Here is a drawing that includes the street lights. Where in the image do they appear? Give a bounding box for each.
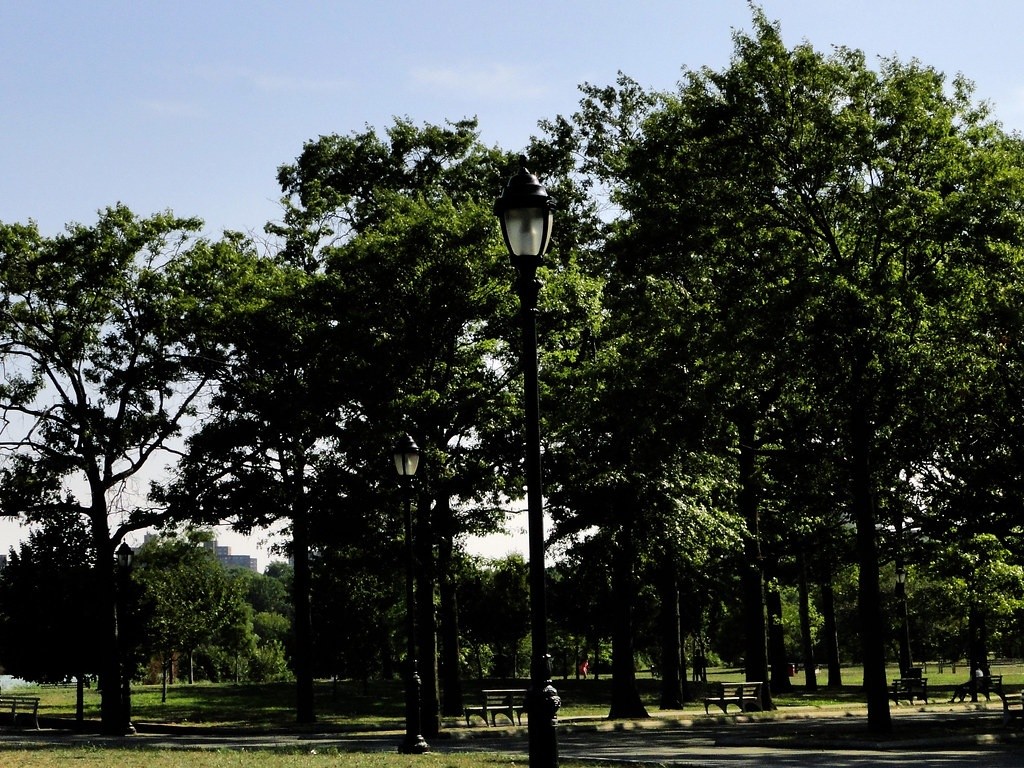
[391,429,431,755]
[491,152,563,768]
[894,566,913,678]
[116,542,137,737]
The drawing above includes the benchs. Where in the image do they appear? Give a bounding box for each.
[888,678,928,706]
[700,681,764,714]
[464,688,529,728]
[0,697,41,732]
[961,674,1004,703]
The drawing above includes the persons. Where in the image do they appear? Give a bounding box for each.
[692,649,707,681]
[580,660,588,679]
[948,662,990,703]
